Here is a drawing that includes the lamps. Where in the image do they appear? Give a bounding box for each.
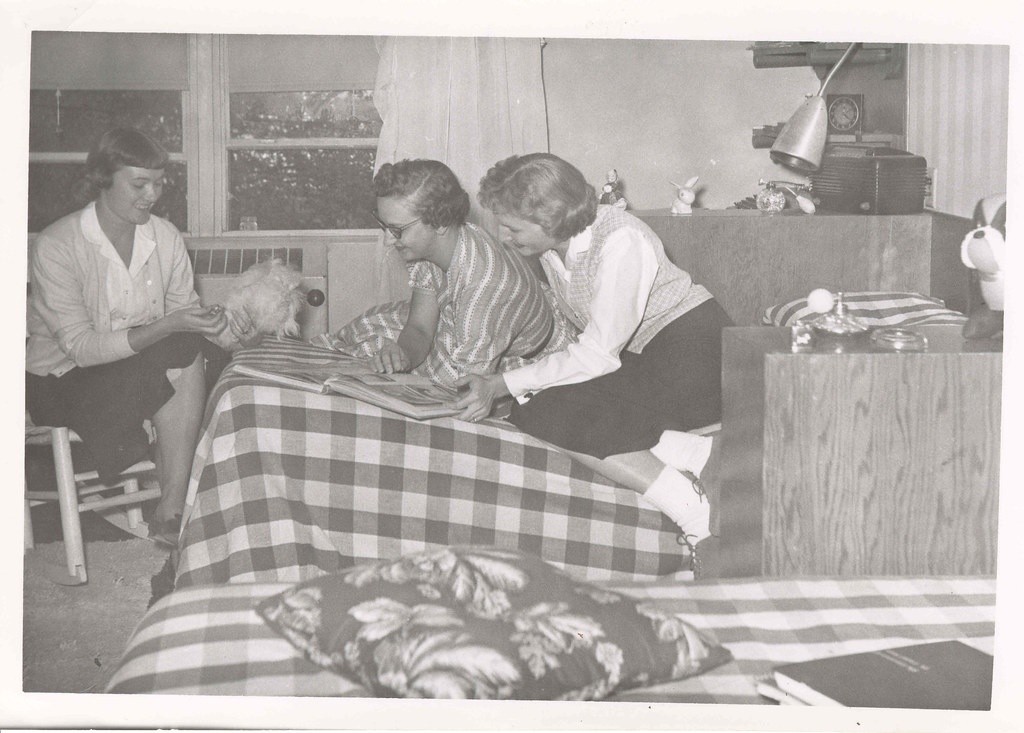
[770,41,861,174]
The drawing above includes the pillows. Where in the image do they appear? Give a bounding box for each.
[250,541,731,699]
[764,297,949,352]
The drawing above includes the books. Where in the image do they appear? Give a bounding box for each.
[758,638,992,711]
[232,358,466,418]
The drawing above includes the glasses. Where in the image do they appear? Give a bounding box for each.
[369,208,423,239]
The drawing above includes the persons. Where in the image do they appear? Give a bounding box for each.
[25,123,254,546]
[440,152,737,587]
[309,156,585,424]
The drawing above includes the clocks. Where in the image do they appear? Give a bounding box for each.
[826,92,866,135]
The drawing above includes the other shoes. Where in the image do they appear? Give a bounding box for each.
[693,433,722,537]
[148,514,181,547]
[679,534,723,580]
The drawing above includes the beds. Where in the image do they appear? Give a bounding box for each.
[110,292,998,710]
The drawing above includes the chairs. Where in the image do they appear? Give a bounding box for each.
[25,230,174,588]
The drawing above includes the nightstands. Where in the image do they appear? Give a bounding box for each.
[714,327,999,574]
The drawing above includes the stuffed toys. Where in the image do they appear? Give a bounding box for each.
[204,255,303,354]
[957,189,1007,340]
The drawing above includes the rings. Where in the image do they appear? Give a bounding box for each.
[474,418,477,422]
[242,329,248,334]
